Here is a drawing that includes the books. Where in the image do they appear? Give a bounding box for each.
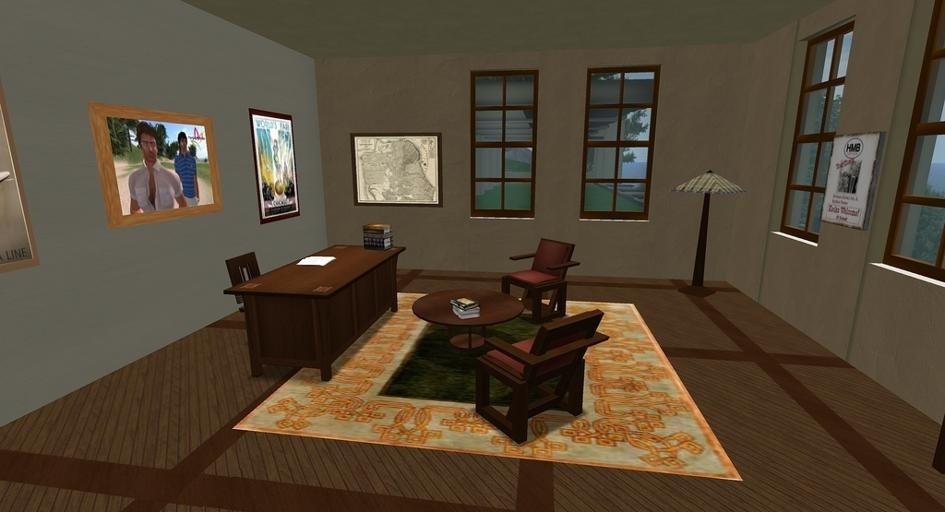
[363,223,393,250]
[295,255,336,266]
[450,296,480,319]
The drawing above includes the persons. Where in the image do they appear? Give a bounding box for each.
[126,121,199,214]
